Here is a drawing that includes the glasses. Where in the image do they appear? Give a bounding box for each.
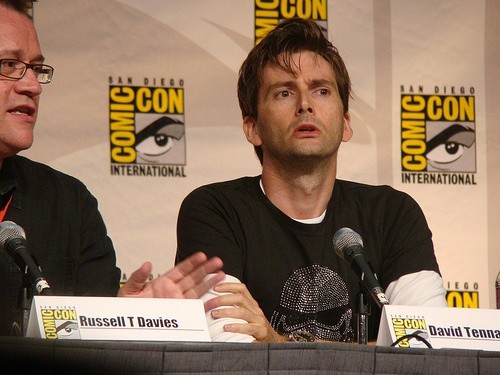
[0,59,54,84]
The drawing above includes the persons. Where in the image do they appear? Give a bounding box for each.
[0,0,226,300]
[176,19,447,344]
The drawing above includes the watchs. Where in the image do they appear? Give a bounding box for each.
[291,329,316,343]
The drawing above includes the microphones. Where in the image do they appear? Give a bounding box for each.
[0,221,52,295]
[332,227,390,309]
